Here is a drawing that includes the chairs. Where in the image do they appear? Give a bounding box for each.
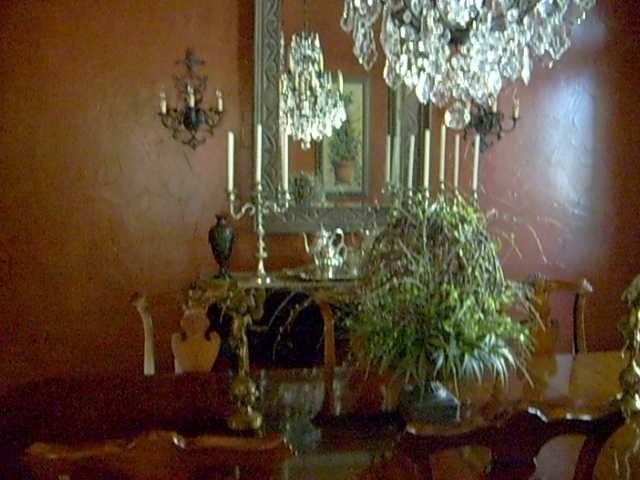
[22,430,296,478]
[132,277,254,373]
[509,275,594,352]
[396,405,625,479]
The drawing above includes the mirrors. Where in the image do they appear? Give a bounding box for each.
[254,0,432,237]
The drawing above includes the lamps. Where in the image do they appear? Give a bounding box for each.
[340,1,596,154]
[158,49,228,149]
[286,0,348,152]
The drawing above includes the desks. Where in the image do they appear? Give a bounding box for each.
[0,351,640,479]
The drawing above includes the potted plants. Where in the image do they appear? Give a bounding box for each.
[272,180,567,426]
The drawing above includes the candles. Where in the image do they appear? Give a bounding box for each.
[280,122,289,191]
[254,122,262,184]
[384,125,480,189]
[227,131,235,191]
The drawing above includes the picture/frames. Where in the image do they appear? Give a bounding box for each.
[315,74,368,198]
